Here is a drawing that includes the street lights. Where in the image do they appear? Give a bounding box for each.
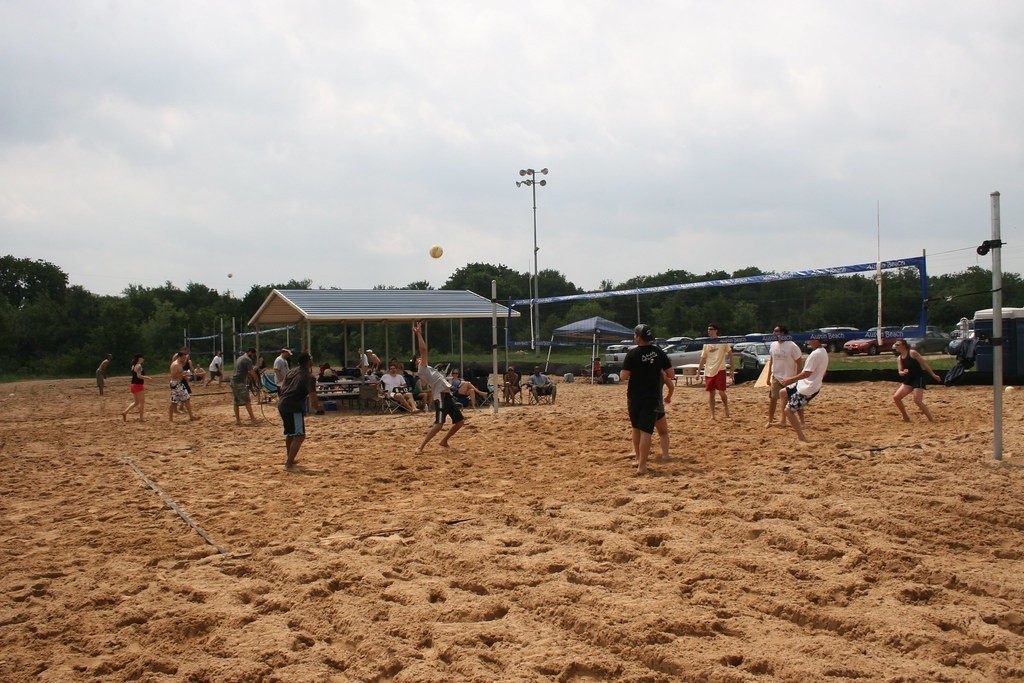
[515,167,549,357]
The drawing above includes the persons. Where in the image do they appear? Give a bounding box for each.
[273,346,293,426]
[769,329,828,443]
[696,323,735,420]
[763,325,802,429]
[96,354,112,395]
[277,352,323,472]
[503,367,518,406]
[356,348,369,371]
[893,340,941,422]
[627,374,674,460]
[409,322,465,455]
[366,357,488,413]
[527,366,556,405]
[122,354,153,422]
[168,347,223,422]
[366,349,381,371]
[620,324,675,474]
[230,348,258,425]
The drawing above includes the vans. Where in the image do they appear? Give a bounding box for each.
[900,324,939,334]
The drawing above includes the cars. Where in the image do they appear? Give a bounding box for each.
[804,327,860,354]
[891,331,950,356]
[843,326,903,354]
[739,341,774,371]
[947,329,975,359]
[605,333,768,368]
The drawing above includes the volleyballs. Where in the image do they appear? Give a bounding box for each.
[324,369,332,376]
[227,274,232,278]
[1005,386,1015,392]
[429,245,443,258]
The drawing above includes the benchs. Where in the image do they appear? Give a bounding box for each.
[316,390,360,409]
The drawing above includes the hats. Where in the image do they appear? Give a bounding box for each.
[282,349,293,355]
[635,324,655,340]
[409,355,418,372]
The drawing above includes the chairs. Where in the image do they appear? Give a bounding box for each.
[497,372,523,406]
[528,372,551,405]
[380,381,411,415]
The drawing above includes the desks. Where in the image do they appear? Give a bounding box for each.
[317,382,379,410]
[674,374,707,386]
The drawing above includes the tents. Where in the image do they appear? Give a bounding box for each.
[544,316,635,385]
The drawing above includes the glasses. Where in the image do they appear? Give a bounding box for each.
[453,373,458,374]
[508,370,513,372]
